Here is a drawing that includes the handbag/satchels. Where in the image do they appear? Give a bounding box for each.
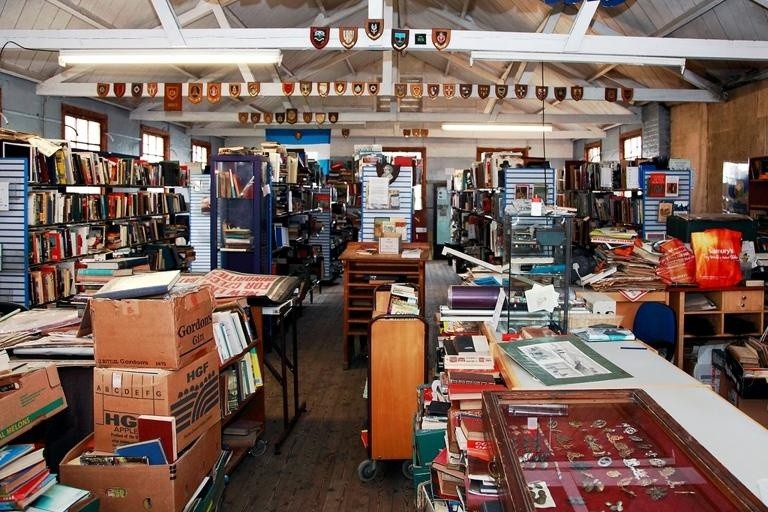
[692,230,743,288]
[659,239,695,286]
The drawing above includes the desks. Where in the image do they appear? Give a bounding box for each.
[478,321,768,511]
[261,282,306,456]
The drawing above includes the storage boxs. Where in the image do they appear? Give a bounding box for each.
[0,365,68,447]
[57,281,222,511]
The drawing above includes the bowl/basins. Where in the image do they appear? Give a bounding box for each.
[653,156,670,170]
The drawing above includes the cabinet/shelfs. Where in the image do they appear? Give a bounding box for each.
[337,242,433,369]
[665,286,768,369]
[450,169,557,275]
[214,305,265,477]
[557,158,691,257]
[0,158,192,309]
[747,154,768,251]
[359,282,430,462]
[211,155,324,304]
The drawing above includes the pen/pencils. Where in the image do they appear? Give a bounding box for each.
[620,347,648,349]
[356,249,373,252]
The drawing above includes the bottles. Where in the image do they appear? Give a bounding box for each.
[331,159,344,170]
[740,253,752,280]
[531,193,541,216]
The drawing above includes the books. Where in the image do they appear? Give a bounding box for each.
[356,217,432,259]
[2,270,299,508]
[361,275,421,450]
[2,140,196,306]
[215,142,363,275]
[446,151,765,394]
[415,304,509,508]
